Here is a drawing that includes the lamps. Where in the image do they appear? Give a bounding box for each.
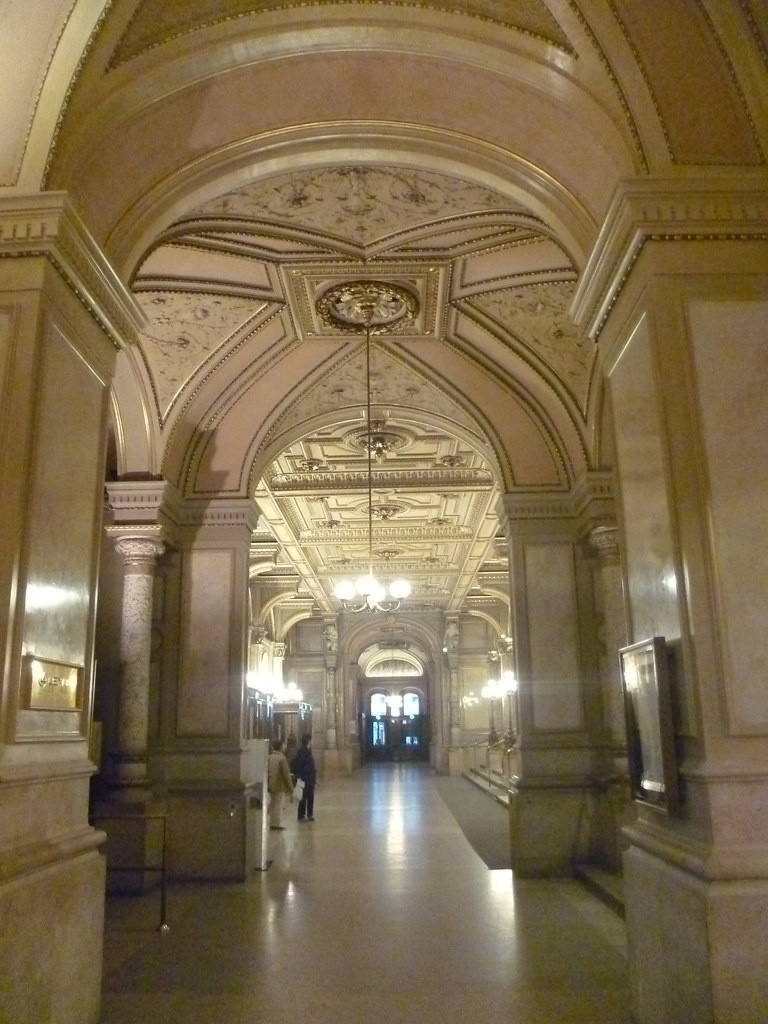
[316,281,418,613]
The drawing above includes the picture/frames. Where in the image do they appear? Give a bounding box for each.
[616,635,683,817]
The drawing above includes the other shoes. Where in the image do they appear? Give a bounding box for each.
[298,816,315,822]
[270,826,284,830]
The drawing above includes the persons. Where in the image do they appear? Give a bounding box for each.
[268,740,294,831]
[294,733,317,821]
